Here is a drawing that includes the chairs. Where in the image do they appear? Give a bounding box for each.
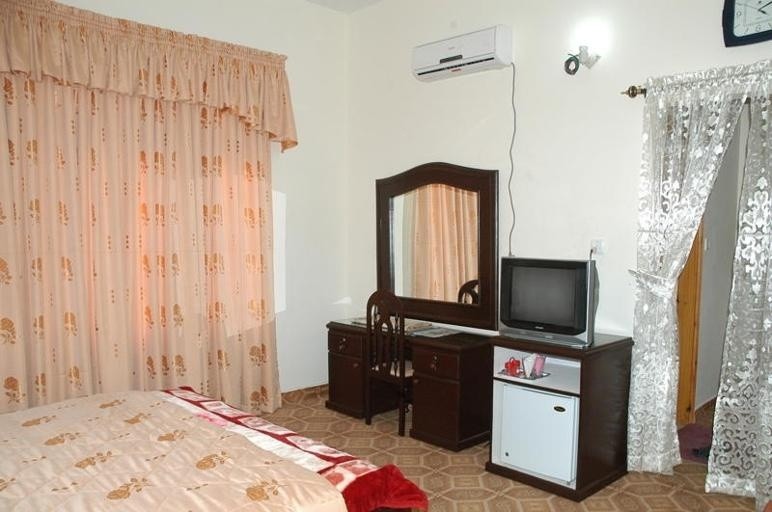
[455,278,481,307]
[365,288,413,434]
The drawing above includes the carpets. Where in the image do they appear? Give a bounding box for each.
[673,422,713,461]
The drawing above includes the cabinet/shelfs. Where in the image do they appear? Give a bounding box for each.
[485,334,633,504]
[324,320,367,418]
[410,322,492,451]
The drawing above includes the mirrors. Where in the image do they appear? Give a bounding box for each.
[376,160,499,332]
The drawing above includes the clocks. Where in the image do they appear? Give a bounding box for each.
[722,1,772,47]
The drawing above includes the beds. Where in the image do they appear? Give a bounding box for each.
[0,385,428,512]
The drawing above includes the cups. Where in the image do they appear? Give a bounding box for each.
[534,354,546,378]
[522,354,535,379]
[505,360,519,376]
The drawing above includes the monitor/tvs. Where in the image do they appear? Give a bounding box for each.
[498,256,600,350]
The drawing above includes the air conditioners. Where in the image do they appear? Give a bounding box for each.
[411,22,510,84]
